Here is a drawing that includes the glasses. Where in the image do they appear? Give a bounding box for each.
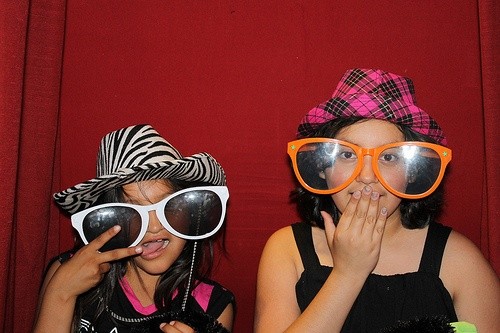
[70,185,229,253]
[286,137,455,200]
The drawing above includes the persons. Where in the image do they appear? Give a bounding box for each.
[34,124,238,333]
[253,69,500,333]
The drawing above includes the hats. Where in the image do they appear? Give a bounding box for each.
[295,68,449,148]
[52,123,226,215]
